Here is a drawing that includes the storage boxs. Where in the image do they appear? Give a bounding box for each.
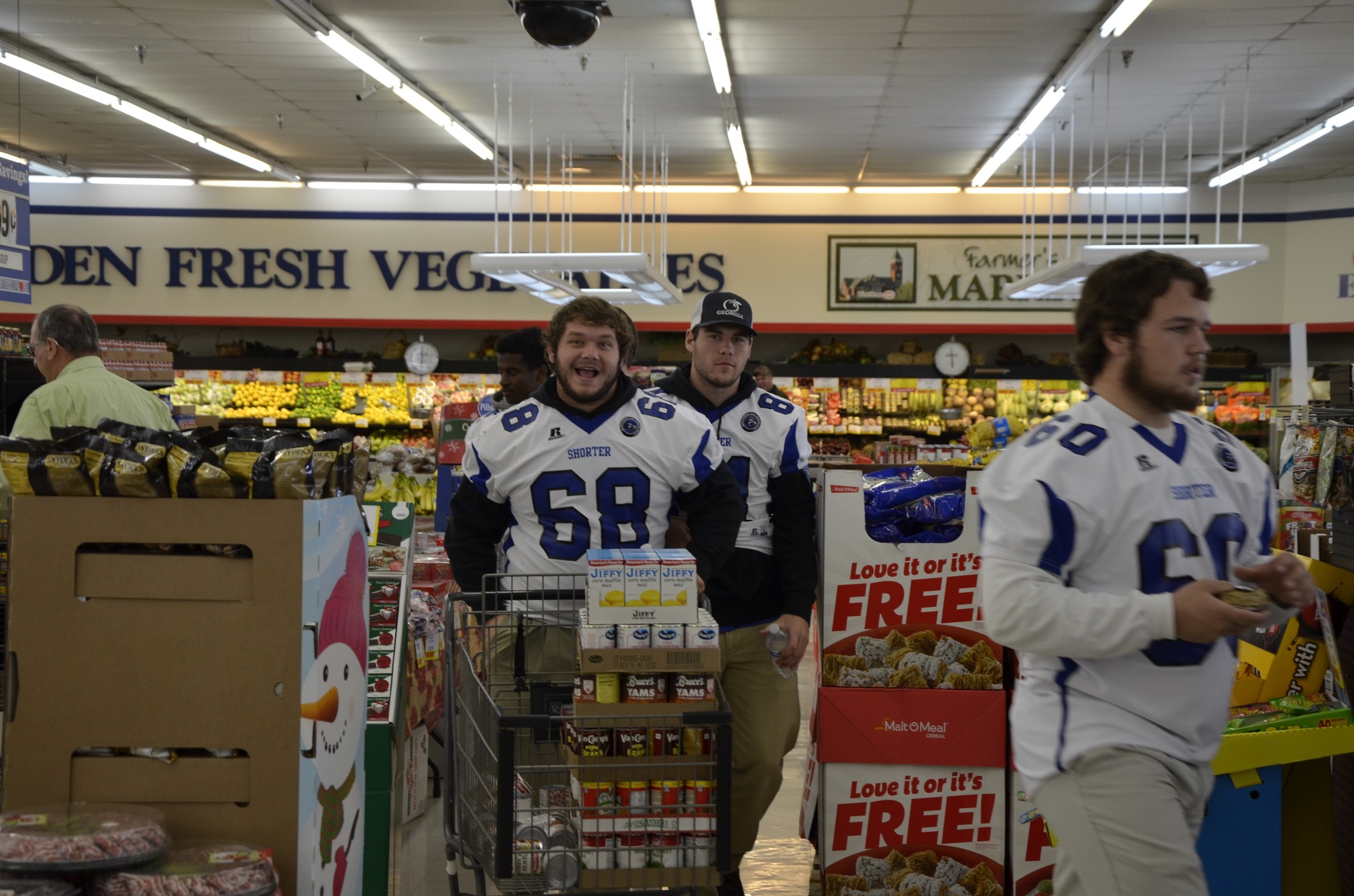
[95,337,174,382]
[561,548,720,891]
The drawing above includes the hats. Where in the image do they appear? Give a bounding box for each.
[691,290,758,339]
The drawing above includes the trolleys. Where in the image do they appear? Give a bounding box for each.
[443,572,735,896]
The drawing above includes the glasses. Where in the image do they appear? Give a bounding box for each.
[24,336,59,358]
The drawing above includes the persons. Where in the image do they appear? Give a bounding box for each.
[976,249,1317,896]
[444,291,820,896]
[8,304,180,439]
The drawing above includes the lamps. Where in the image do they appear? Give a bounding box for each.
[0,0,1354,309]
